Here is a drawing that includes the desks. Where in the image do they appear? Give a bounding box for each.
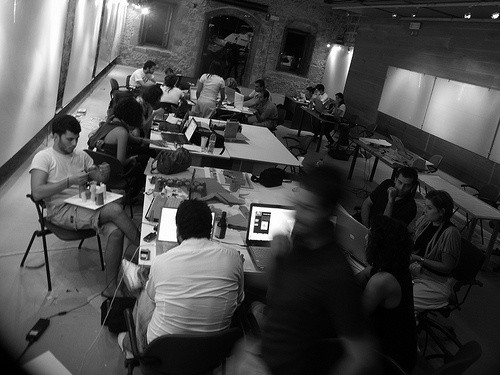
[138,82,500,273]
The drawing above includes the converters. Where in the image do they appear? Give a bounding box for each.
[25,318,50,342]
[143,233,157,243]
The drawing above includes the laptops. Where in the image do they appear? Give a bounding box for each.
[225,87,250,112]
[155,207,216,258]
[158,109,199,145]
[190,86,198,104]
[146,169,196,222]
[335,205,370,267]
[245,203,297,272]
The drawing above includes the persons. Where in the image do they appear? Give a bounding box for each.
[30,115,140,298]
[354,167,418,226]
[225,77,278,128]
[220,42,239,78]
[350,214,417,375]
[96,85,168,206]
[252,165,362,375]
[406,190,462,314]
[117,199,244,360]
[129,60,225,119]
[301,84,346,146]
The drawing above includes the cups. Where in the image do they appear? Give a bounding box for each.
[200,136,208,152]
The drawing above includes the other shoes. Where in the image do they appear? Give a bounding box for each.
[326,142,336,148]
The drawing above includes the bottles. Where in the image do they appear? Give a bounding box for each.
[215,211,227,239]
[206,131,217,154]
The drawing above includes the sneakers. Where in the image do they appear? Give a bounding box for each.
[117,332,134,360]
[122,258,145,292]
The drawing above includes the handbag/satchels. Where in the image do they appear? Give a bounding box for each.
[193,126,225,148]
[150,146,192,175]
[251,168,293,187]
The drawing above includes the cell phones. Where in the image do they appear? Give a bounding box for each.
[140,249,150,260]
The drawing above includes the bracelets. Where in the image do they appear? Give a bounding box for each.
[421,257,424,265]
[67,177,69,188]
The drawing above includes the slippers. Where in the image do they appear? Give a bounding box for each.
[101,290,126,300]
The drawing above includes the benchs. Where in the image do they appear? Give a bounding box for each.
[331,110,376,160]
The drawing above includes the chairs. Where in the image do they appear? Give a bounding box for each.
[110,76,134,100]
[275,135,314,175]
[20,194,105,292]
[272,108,286,137]
[453,185,500,245]
[122,308,243,375]
[83,149,143,218]
[415,314,482,375]
[419,155,443,195]
[418,239,488,316]
